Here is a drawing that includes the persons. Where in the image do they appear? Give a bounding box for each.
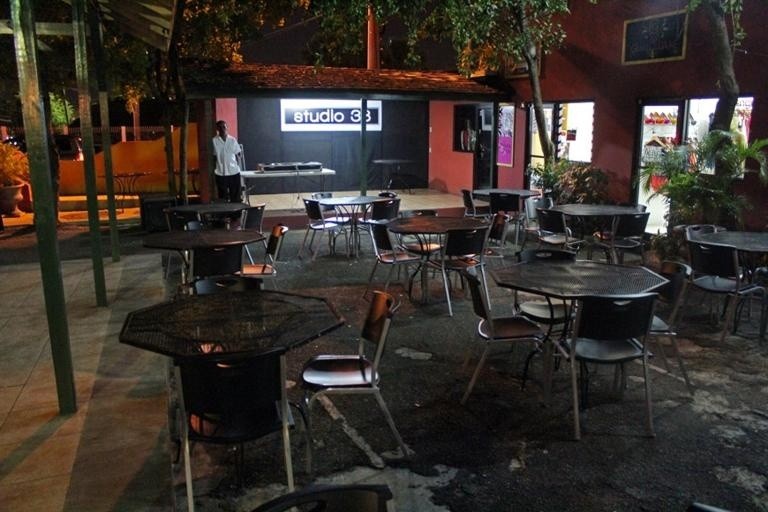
[210,119,244,231]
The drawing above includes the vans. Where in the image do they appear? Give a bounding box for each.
[4,134,83,162]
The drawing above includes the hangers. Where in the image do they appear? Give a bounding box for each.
[644,130,664,147]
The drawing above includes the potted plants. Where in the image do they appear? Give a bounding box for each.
[0,144,30,217]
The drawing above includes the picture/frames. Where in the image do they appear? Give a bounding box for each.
[504,38,542,79]
[496,102,515,168]
[620,9,690,66]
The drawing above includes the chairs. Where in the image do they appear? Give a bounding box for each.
[675,222,766,350]
[420,226,493,317]
[458,264,553,409]
[120,198,653,289]
[620,259,698,397]
[514,248,579,391]
[170,349,316,512]
[553,292,661,441]
[301,290,413,464]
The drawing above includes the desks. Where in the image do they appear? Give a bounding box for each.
[98,171,152,213]
[120,290,324,492]
[162,168,200,194]
[373,159,417,194]
[240,167,336,206]
